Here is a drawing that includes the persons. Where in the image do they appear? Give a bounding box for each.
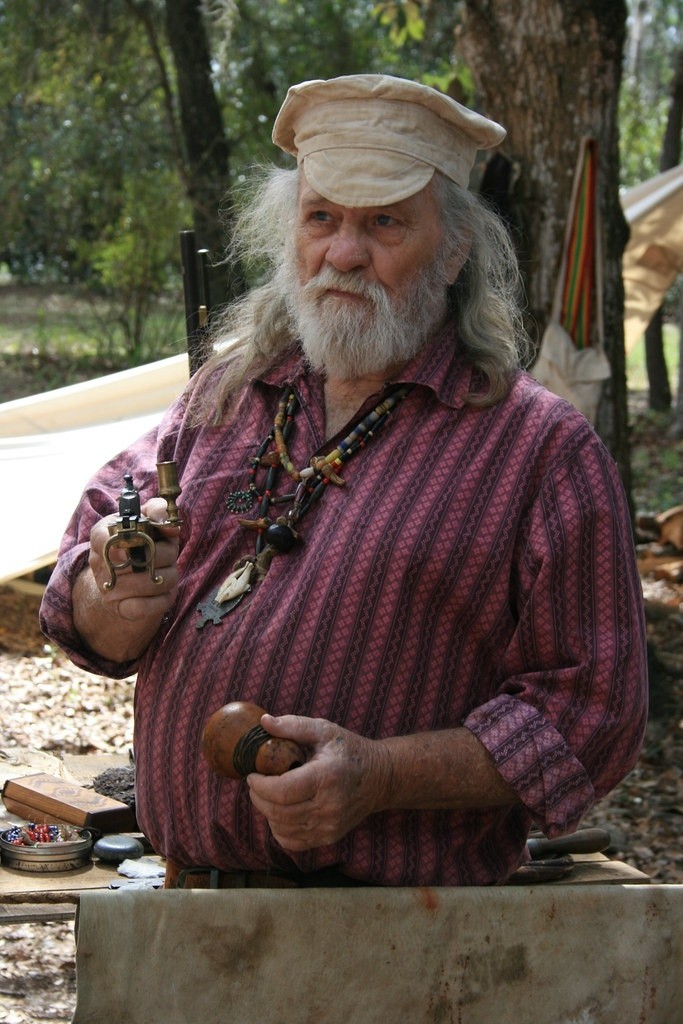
[37,67,650,891]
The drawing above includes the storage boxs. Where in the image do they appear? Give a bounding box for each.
[0,773,130,839]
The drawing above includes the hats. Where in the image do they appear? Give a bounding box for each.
[270,73,508,208]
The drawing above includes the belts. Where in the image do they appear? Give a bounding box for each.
[164,860,247,889]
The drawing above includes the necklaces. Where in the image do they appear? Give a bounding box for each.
[191,370,419,632]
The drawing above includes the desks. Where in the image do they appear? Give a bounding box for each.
[0,787,650,923]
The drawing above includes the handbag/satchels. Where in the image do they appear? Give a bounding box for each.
[531,321,611,429]
